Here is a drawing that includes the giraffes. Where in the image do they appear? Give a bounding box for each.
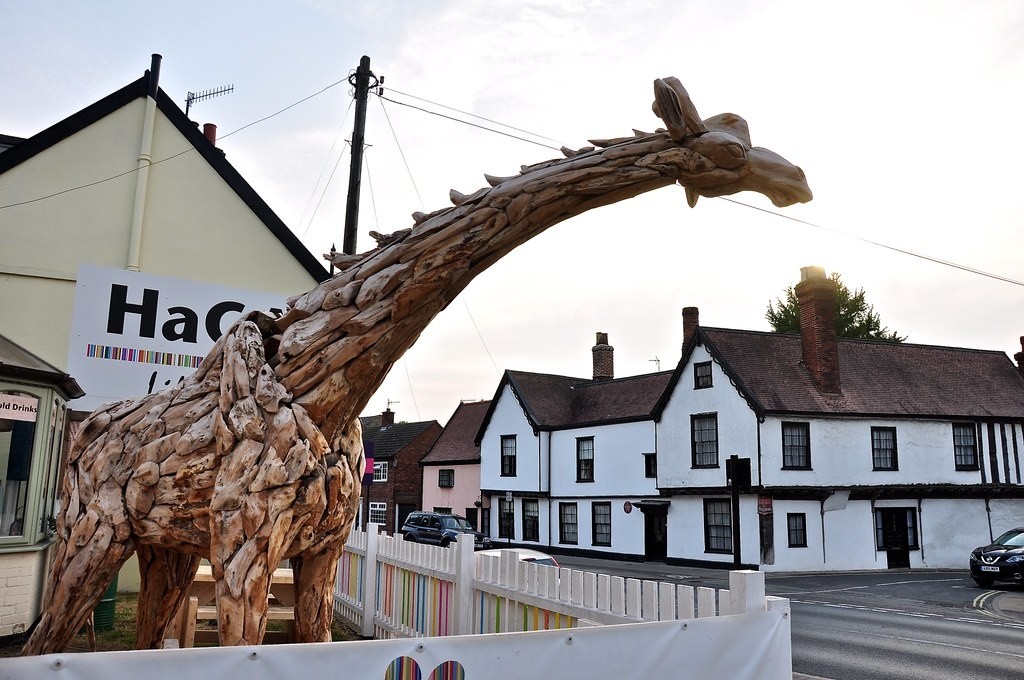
[19,74,811,657]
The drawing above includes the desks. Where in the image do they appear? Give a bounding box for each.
[165,566,295,648]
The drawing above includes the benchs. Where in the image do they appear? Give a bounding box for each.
[185,596,295,648]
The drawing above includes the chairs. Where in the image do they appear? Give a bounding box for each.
[449,520,455,527]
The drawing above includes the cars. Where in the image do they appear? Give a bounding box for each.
[969,526,1024,588]
[474,547,561,586]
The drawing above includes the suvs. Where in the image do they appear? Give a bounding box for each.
[400,510,492,556]
